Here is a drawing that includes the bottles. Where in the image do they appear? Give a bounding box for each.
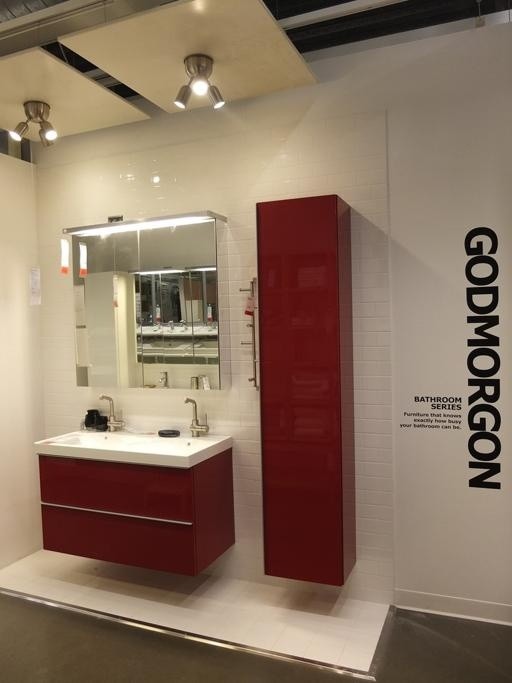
[206,304,213,323]
[155,304,162,321]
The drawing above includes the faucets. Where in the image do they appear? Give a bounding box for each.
[191,377,198,389]
[182,397,210,437]
[98,394,124,432]
[159,371,167,388]
[180,319,188,330]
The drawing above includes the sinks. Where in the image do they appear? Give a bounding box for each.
[33,429,233,471]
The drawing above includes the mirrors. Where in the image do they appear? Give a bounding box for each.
[61,210,223,390]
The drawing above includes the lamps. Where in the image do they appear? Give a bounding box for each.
[173,53,225,110]
[11,101,58,149]
